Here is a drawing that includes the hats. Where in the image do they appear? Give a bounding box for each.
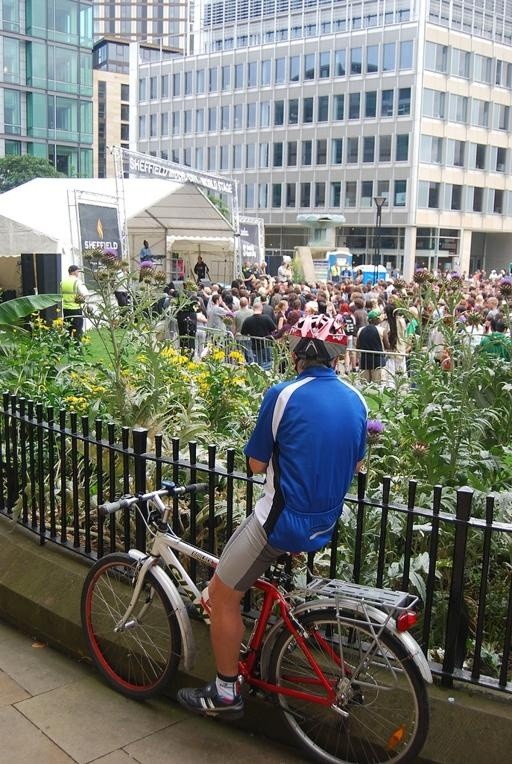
[368,309,379,320]
[68,266,79,273]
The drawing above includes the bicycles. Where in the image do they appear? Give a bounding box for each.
[79,482,433,764]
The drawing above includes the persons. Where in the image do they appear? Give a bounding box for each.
[59,239,512,387]
[176,312,369,719]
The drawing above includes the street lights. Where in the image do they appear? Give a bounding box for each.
[374,197,386,282]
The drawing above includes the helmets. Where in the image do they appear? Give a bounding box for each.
[288,313,347,361]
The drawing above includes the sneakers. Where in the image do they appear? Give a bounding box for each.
[186,600,202,621]
[176,684,247,722]
